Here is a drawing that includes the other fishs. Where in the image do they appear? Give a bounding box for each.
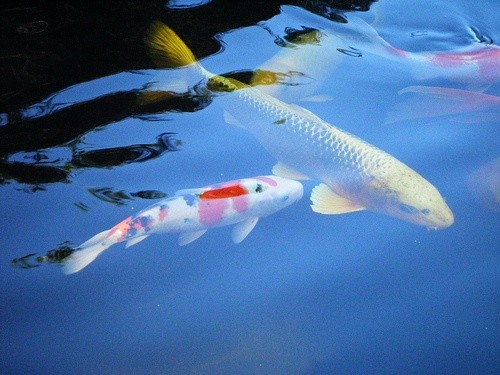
[58,18,500,275]
